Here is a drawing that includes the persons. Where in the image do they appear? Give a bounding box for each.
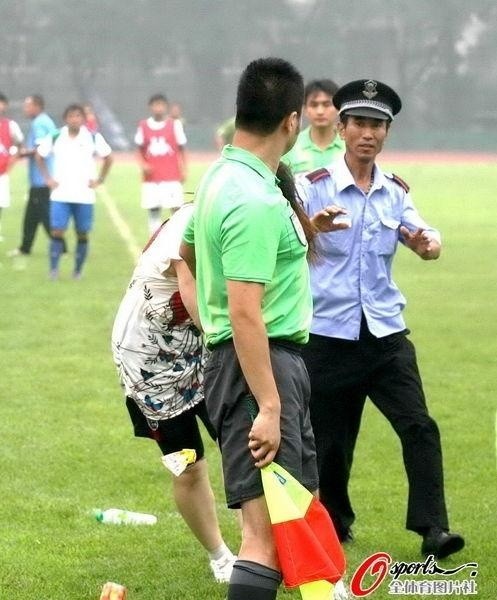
[1,93,187,279]
[215,118,236,150]
[285,78,346,175]
[112,161,298,586]
[178,59,322,599]
[291,81,466,555]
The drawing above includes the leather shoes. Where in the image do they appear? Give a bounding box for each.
[421,530,466,561]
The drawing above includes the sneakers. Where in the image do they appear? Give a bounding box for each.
[210,551,237,585]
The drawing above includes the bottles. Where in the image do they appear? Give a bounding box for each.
[90,509,157,527]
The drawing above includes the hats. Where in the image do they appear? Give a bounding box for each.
[331,78,403,121]
